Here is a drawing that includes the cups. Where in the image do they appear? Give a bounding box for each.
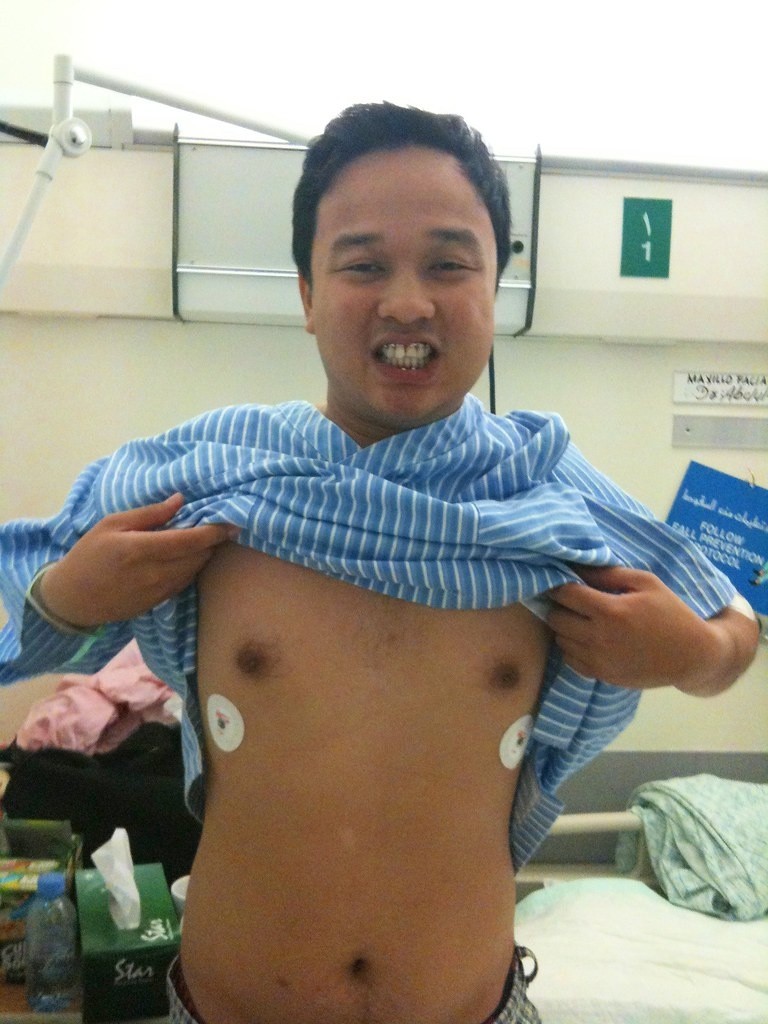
[170,874,192,921]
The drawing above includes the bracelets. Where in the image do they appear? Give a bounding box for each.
[25,564,106,637]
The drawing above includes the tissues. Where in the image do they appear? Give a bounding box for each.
[72,827,183,991]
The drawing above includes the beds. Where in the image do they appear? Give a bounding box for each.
[509,808,768,1024]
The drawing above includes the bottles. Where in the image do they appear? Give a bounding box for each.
[25,872,79,1014]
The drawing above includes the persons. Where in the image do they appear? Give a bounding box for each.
[0,100,757,1024]
